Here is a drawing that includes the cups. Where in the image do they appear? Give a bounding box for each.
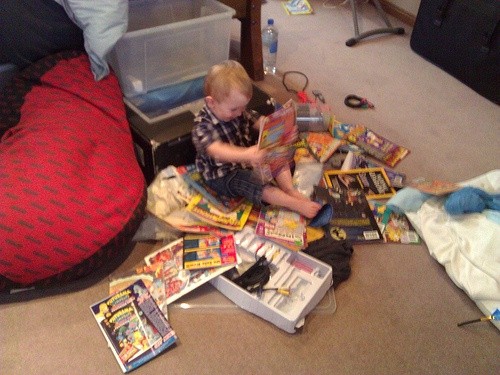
[296,103,332,132]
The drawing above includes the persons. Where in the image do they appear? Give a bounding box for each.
[192,59,323,219]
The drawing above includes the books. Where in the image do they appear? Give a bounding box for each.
[88,98,465,374]
[281,0,314,15]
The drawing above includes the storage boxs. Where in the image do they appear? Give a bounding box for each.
[107,0,236,101]
[210,226,333,333]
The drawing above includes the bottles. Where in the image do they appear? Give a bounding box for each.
[261,19,279,76]
